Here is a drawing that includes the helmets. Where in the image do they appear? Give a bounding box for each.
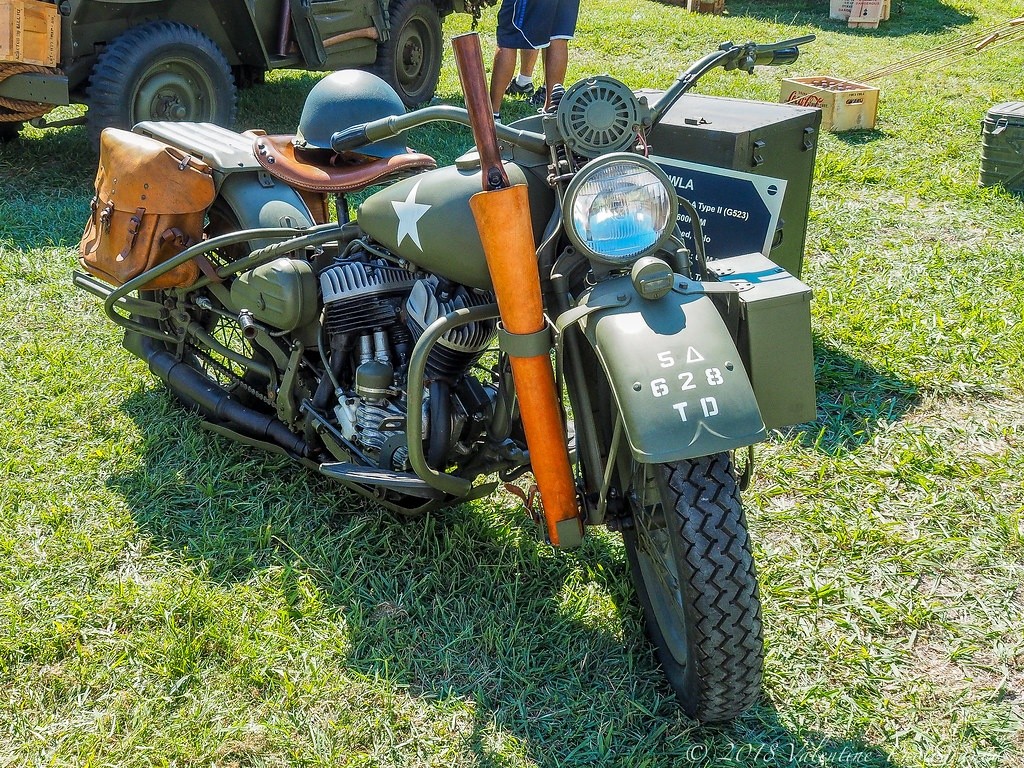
[290,69,409,160]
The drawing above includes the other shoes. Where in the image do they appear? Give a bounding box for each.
[494,113,502,125]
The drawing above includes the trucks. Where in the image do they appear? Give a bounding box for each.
[0,0,463,159]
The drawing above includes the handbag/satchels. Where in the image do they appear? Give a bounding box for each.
[80,127,215,291]
[246,127,328,227]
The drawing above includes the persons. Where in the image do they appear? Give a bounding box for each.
[489,0,581,124]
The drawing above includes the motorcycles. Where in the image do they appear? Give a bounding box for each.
[71,34,818,726]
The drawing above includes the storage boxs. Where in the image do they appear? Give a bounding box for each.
[978,102,1024,198]
[686,0,724,16]
[0,0,62,69]
[779,76,880,134]
[828,0,892,29]
[633,88,824,287]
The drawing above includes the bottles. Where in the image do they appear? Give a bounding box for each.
[797,80,861,91]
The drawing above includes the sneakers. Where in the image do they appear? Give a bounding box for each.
[504,76,535,97]
[530,85,547,107]
